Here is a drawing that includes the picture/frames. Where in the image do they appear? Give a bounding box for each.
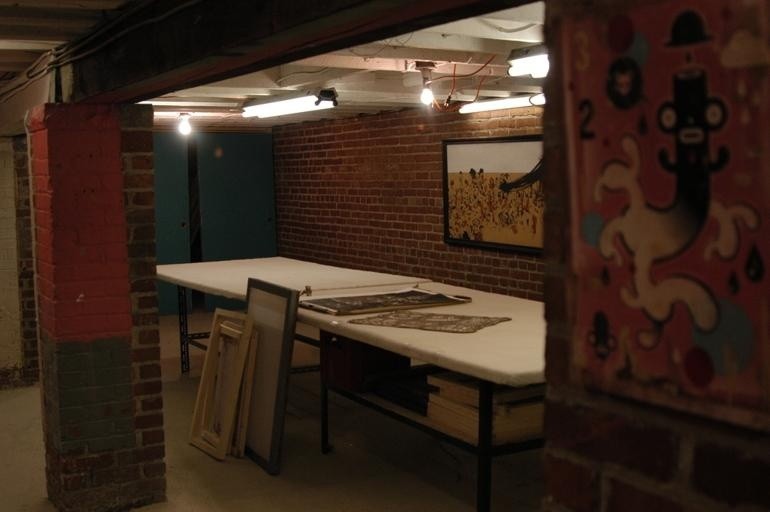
[441,133,548,255]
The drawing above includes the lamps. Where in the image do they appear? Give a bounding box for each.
[240,86,342,119]
[505,42,550,78]
[458,93,546,115]
